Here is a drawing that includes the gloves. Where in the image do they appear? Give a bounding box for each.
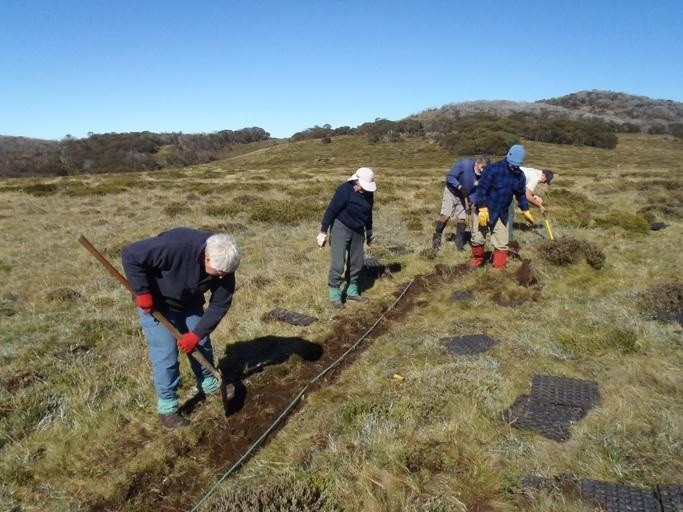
[135,293,153,315]
[316,232,327,248]
[477,206,489,227]
[178,331,200,353]
[366,233,376,247]
[522,210,534,227]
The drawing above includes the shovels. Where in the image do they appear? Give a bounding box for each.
[77,234,230,417]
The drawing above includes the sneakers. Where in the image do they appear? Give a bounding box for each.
[330,299,346,310]
[158,410,192,429]
[346,295,369,304]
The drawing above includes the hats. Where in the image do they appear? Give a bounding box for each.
[543,169,554,186]
[356,167,377,192]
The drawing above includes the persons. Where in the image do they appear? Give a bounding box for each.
[469,144,534,270]
[432,155,490,251]
[122,227,240,432]
[316,167,377,310]
[519,166,553,216]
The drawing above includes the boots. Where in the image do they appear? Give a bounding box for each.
[468,245,485,270]
[492,247,509,270]
[455,222,466,252]
[432,220,446,253]
